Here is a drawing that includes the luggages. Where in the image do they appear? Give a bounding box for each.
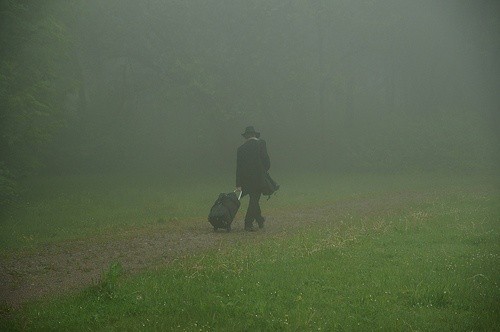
[208,187,242,232]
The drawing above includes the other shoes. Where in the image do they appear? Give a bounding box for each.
[245,225,257,232]
[257,216,264,228]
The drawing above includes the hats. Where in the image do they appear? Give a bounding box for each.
[240,126,260,137]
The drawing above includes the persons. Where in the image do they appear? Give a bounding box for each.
[235,126,271,232]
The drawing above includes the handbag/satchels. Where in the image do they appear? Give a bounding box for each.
[259,174,279,198]
